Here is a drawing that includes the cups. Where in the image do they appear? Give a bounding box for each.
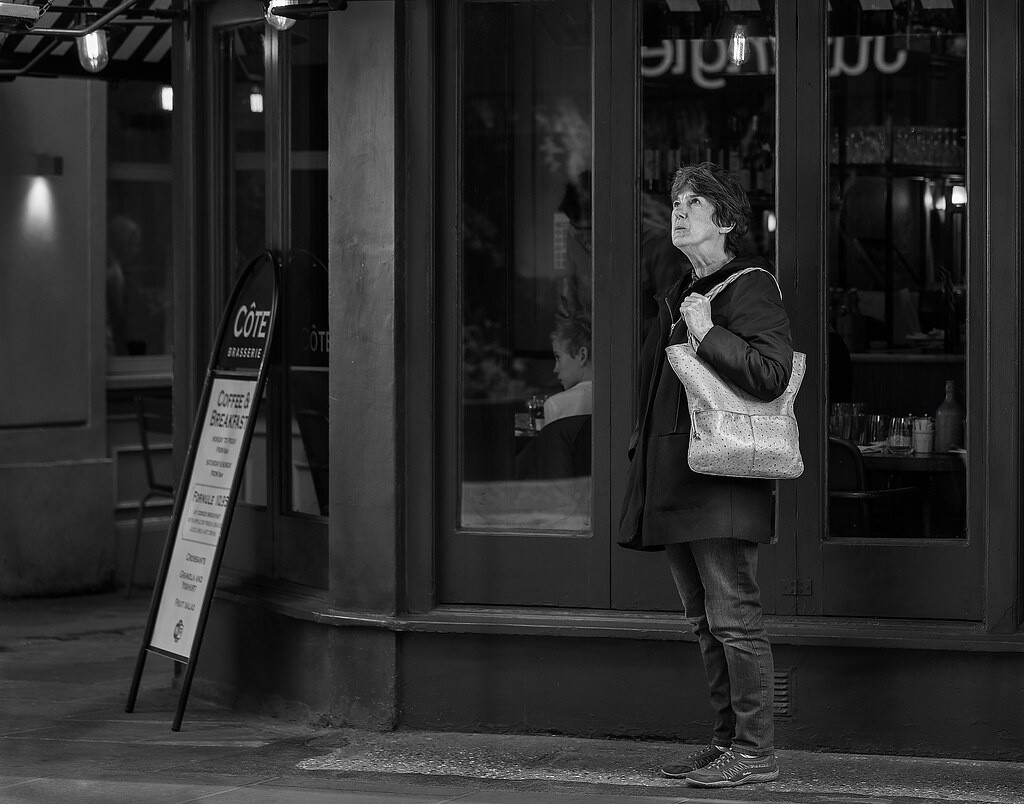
[913,431,933,453]
[527,399,543,432]
[830,402,866,446]
[868,415,912,451]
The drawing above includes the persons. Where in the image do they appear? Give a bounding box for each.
[610,161,809,789]
[542,315,592,425]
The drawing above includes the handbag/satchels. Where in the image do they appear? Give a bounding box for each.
[665,267,806,479]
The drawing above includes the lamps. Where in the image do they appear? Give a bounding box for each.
[74,24,108,73]
[726,23,751,67]
[261,0,347,31]
[935,174,967,214]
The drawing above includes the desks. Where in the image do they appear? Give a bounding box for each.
[859,441,967,539]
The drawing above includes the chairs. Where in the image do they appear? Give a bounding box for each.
[827,436,907,538]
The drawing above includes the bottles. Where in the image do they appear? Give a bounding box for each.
[934,380,966,453]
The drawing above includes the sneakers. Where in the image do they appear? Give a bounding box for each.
[661,744,779,787]
